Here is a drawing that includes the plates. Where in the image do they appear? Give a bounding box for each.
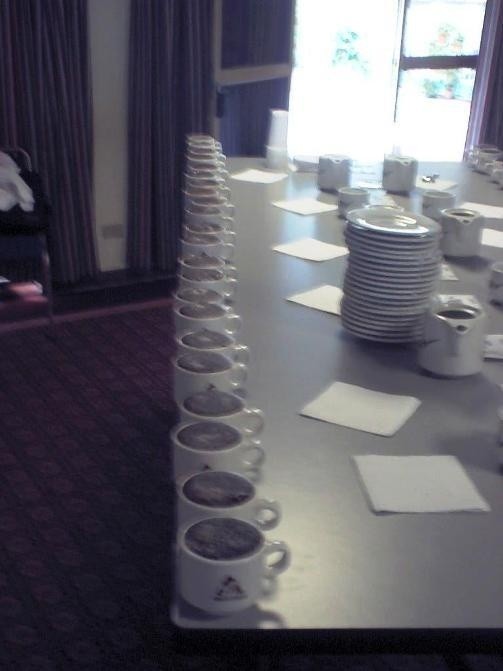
[341,203,441,343]
[484,331,502,359]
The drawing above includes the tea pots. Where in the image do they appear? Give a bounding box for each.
[316,152,352,191]
[421,297,491,379]
[381,152,418,192]
[439,207,483,260]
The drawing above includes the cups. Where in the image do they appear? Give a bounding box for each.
[168,134,240,307]
[175,328,248,363]
[175,351,247,393]
[173,475,281,534]
[173,306,242,336]
[179,389,265,439]
[420,188,455,217]
[488,261,502,306]
[463,140,502,187]
[334,185,371,215]
[176,518,293,609]
[171,423,267,472]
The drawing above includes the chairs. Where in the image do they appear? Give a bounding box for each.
[0,147,57,340]
